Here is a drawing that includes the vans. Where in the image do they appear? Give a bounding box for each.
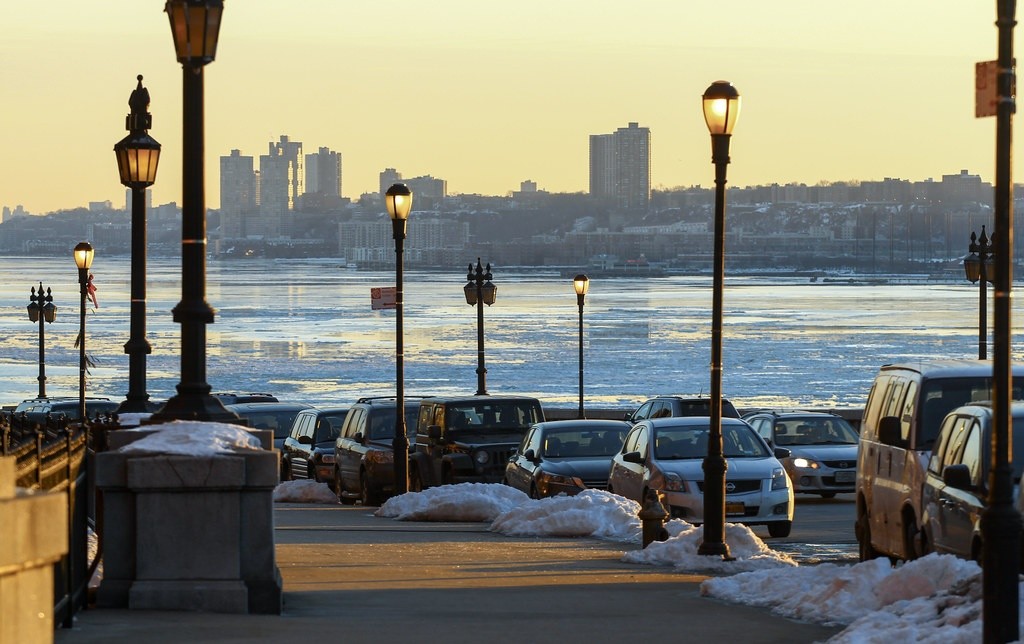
[854,360,1024,567]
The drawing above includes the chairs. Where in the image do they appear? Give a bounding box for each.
[920,398,948,442]
[656,437,676,458]
[695,432,731,457]
[547,437,562,457]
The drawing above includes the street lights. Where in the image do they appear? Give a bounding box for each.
[573,274,589,419]
[464,258,498,396]
[72,243,95,423]
[386,183,413,499]
[964,225,997,360]
[27,282,57,398]
[140,1,238,422]
[114,75,163,414]
[696,80,741,558]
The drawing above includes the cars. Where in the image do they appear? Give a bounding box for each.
[223,402,315,454]
[606,416,795,537]
[503,419,636,499]
[745,414,860,499]
[283,407,351,490]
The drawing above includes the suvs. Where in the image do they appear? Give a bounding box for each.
[919,399,1024,574]
[333,395,437,506]
[410,395,547,493]
[210,392,279,405]
[14,397,119,419]
[624,395,743,425]
